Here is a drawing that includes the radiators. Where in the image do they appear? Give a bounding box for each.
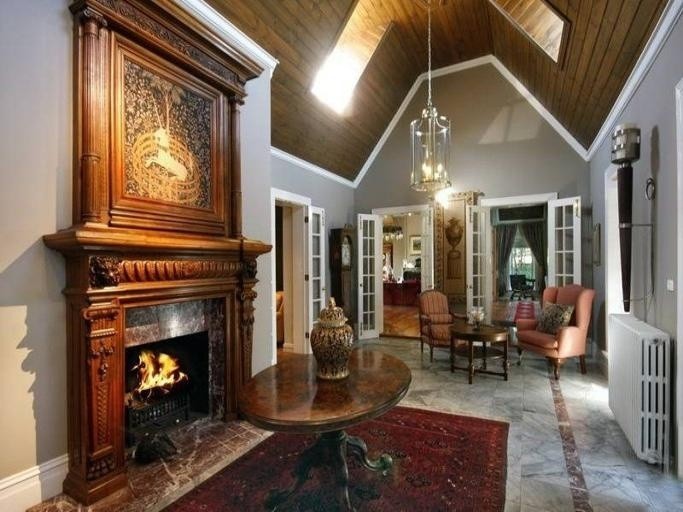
[603,308,672,470]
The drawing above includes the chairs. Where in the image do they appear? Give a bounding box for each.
[511,274,538,299]
[416,287,469,361]
[514,285,594,380]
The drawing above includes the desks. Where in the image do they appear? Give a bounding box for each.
[235,345,413,510]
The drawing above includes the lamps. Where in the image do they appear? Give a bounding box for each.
[408,0,453,193]
[609,121,654,313]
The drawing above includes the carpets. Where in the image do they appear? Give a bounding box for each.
[160,410,513,511]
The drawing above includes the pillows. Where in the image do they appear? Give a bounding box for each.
[536,301,572,336]
